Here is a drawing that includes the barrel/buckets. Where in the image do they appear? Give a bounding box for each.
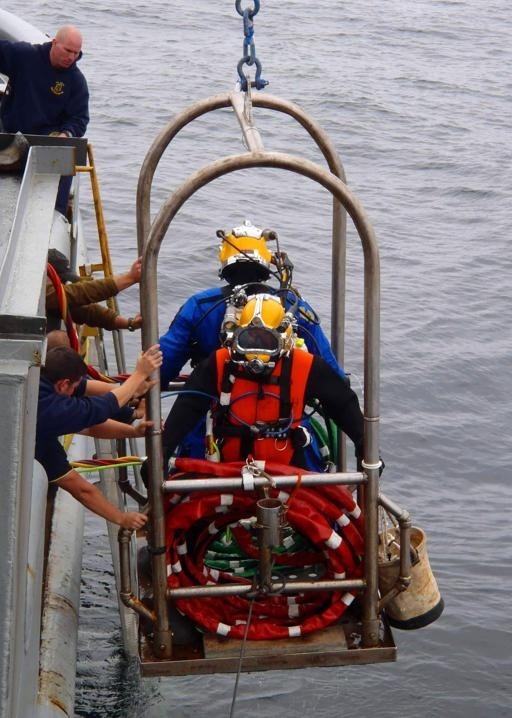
[375,525,445,632]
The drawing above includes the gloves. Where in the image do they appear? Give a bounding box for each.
[354,436,384,477]
[140,434,177,489]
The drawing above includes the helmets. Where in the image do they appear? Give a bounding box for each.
[219,220,272,285]
[219,284,294,374]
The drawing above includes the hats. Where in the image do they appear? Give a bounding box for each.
[48,249,81,283]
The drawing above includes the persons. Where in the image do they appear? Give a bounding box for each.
[35,249,164,532]
[158,219,350,476]
[140,282,386,490]
[0,25,89,217]
